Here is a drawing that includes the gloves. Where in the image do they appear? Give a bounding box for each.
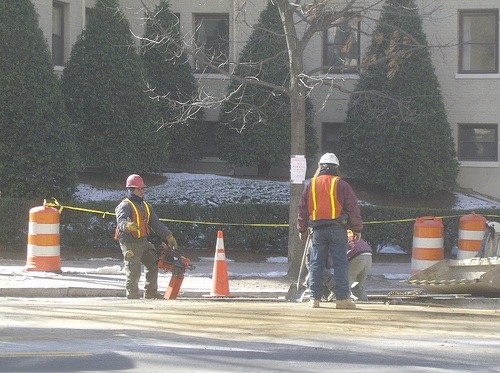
[126,221,139,232]
[166,234,177,250]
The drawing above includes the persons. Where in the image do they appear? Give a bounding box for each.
[328,230,372,301]
[297,152,363,309]
[115,175,177,299]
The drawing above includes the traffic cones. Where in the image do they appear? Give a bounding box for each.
[201,230,237,298]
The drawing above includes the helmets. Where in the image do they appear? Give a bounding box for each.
[125,174,147,188]
[319,153,339,167]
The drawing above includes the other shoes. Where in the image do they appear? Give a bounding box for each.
[309,299,320,308]
[144,291,164,299]
[336,298,356,309]
